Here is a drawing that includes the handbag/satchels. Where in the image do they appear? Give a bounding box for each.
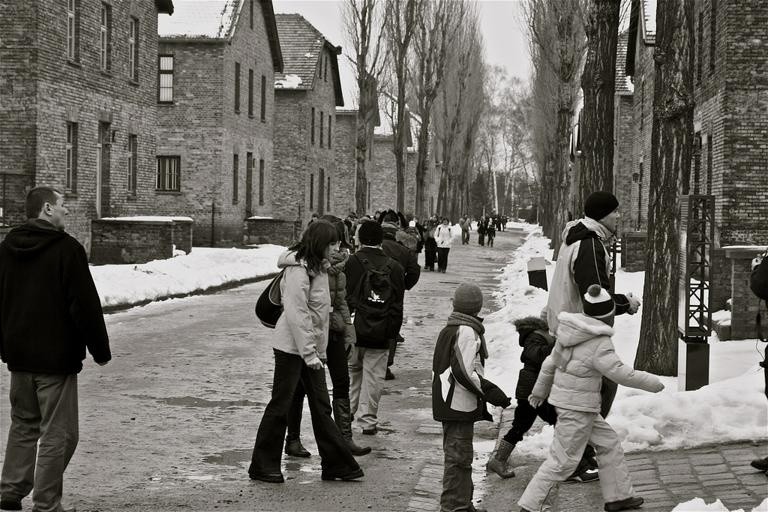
[255,265,312,328]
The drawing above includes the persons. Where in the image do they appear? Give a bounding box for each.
[546,191,640,481]
[430,280,512,512]
[517,284,664,512]
[0,186,112,510]
[459,213,496,247]
[487,304,556,483]
[245,212,371,484]
[419,213,455,273]
[344,208,421,436]
[490,212,509,232]
[748,245,768,476]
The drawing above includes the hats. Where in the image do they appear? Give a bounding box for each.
[453,283,482,313]
[381,209,400,235]
[358,221,383,246]
[584,192,619,221]
[582,284,616,318]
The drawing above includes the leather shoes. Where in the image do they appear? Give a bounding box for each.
[321,469,365,481]
[249,474,283,482]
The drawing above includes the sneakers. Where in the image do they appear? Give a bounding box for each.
[604,497,644,512]
[383,368,394,380]
[561,466,599,483]
[751,457,768,470]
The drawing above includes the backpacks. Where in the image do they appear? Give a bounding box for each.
[353,251,402,349]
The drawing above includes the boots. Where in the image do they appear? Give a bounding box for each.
[285,404,311,457]
[332,398,372,456]
[487,439,515,479]
